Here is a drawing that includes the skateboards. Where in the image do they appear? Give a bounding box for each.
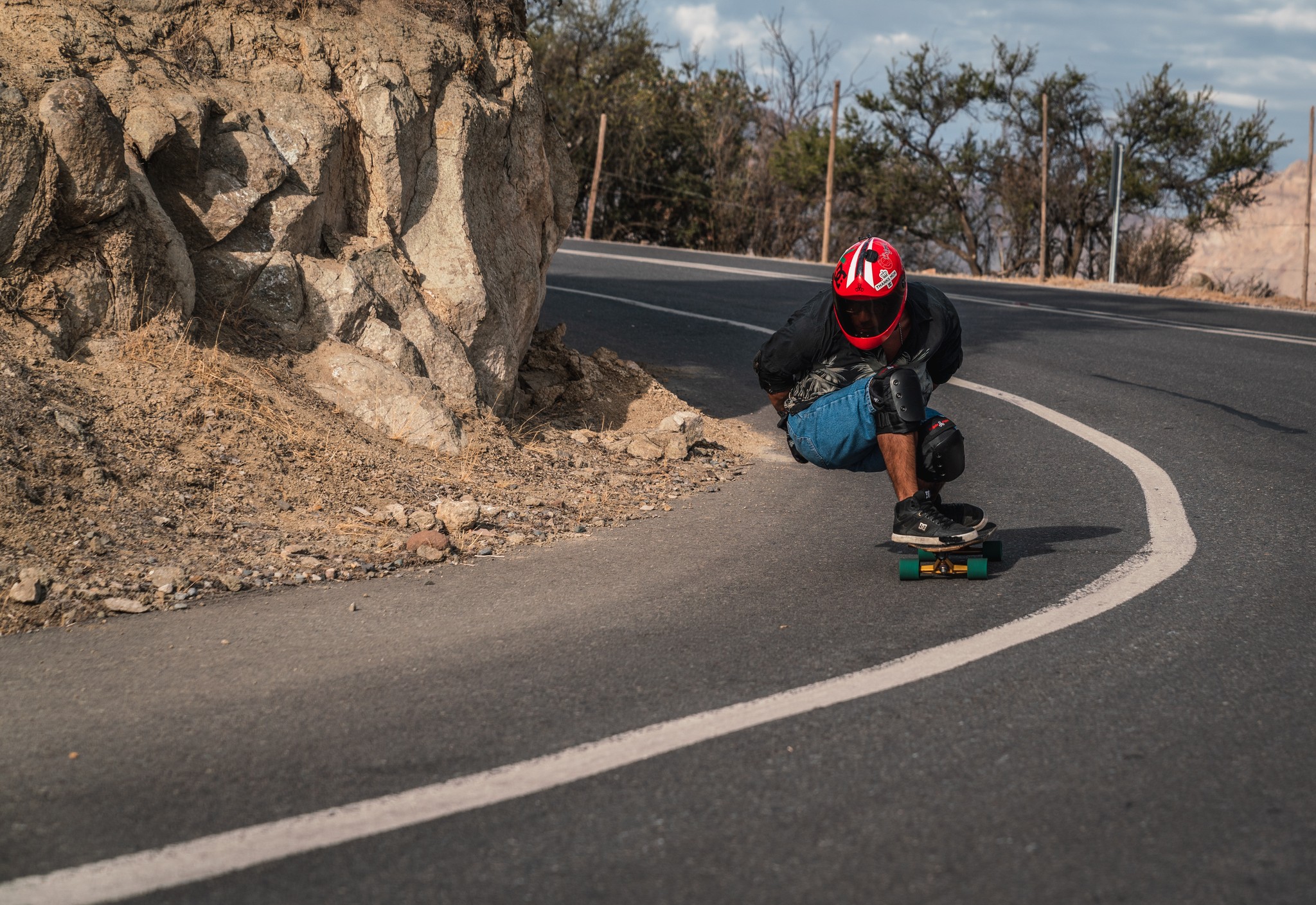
[899,522,1003,581]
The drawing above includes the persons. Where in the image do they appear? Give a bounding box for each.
[752,237,989,547]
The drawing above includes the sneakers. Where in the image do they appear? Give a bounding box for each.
[891,490,987,544]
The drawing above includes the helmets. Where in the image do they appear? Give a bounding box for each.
[832,236,908,351]
